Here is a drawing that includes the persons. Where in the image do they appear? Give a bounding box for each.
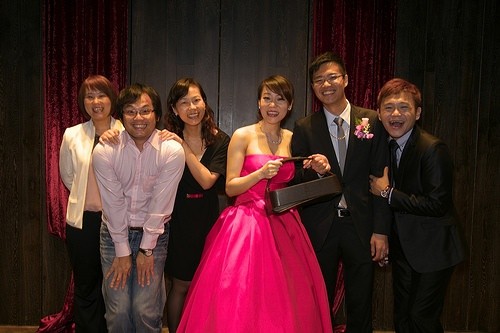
[92,82,186,333]
[290,51,389,333]
[176,73,333,333]
[368,78,460,333]
[59,74,125,333]
[98,77,231,333]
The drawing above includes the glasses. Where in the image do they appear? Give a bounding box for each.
[121,109,155,116]
[313,73,346,86]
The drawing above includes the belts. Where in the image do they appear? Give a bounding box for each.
[335,207,351,218]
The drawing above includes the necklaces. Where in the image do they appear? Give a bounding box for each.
[258,123,282,144]
[328,125,349,140]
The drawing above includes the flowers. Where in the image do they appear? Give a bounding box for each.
[353,115,374,142]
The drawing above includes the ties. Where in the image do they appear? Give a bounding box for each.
[334,117,347,176]
[388,139,400,189]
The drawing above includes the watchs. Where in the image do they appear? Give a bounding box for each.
[139,248,154,257]
[381,186,391,198]
